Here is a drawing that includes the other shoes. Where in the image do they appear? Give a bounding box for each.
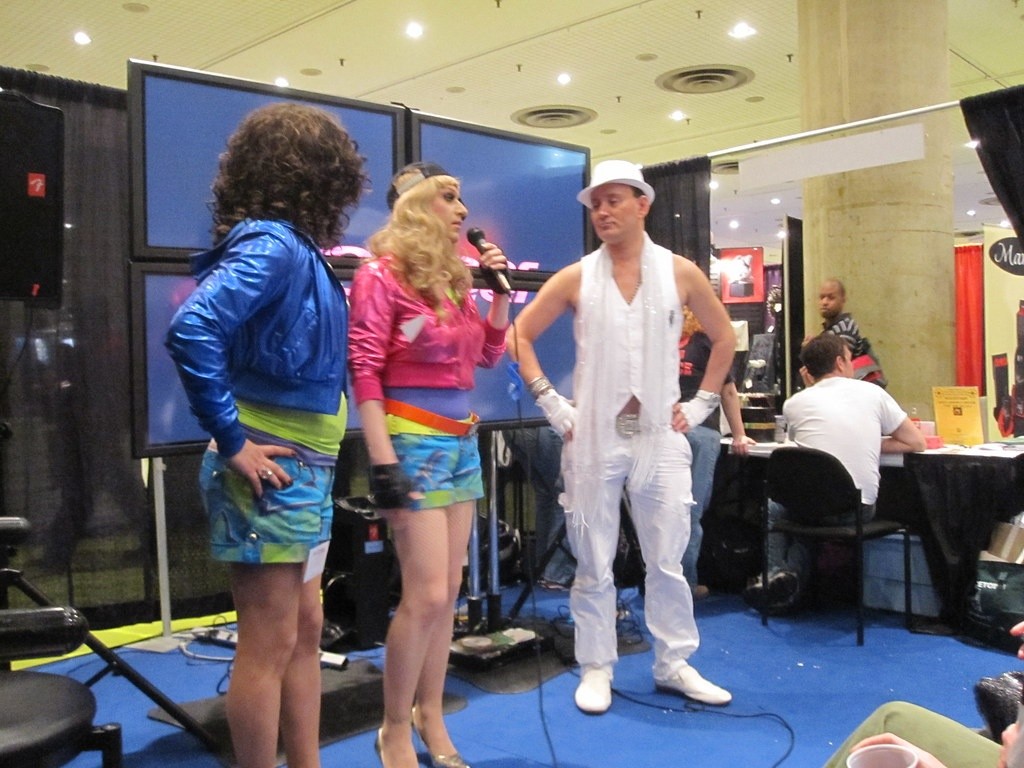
[694,585,709,600]
[746,570,800,615]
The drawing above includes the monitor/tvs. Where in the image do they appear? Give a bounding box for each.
[125,58,594,457]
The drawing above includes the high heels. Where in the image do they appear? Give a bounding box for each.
[375,728,421,768]
[411,707,471,768]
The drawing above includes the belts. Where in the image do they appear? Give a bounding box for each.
[384,397,481,439]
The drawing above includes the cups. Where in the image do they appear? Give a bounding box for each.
[846,743,919,768]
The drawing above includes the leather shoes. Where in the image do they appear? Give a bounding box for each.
[654,667,732,705]
[574,670,612,714]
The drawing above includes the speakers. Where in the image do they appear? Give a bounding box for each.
[320,495,404,652]
[0,89,66,303]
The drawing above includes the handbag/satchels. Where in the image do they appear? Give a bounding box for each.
[957,556,1024,654]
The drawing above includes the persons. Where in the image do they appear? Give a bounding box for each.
[503,161,738,708]
[161,100,373,768]
[799,280,864,388]
[822,621,1024,768]
[743,332,926,614]
[348,160,511,768]
[679,300,757,600]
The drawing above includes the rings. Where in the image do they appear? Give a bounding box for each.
[259,467,272,479]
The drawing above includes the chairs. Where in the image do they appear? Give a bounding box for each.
[0,516,122,767]
[760,447,911,646]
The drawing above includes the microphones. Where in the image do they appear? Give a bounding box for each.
[466,225,514,296]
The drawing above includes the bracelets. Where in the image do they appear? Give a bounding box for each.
[696,389,722,404]
[526,375,552,397]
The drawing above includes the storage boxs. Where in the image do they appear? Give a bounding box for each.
[863,531,942,618]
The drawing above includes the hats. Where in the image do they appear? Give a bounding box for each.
[577,160,656,210]
[388,161,464,212]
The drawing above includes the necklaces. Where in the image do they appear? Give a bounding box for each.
[627,274,641,304]
